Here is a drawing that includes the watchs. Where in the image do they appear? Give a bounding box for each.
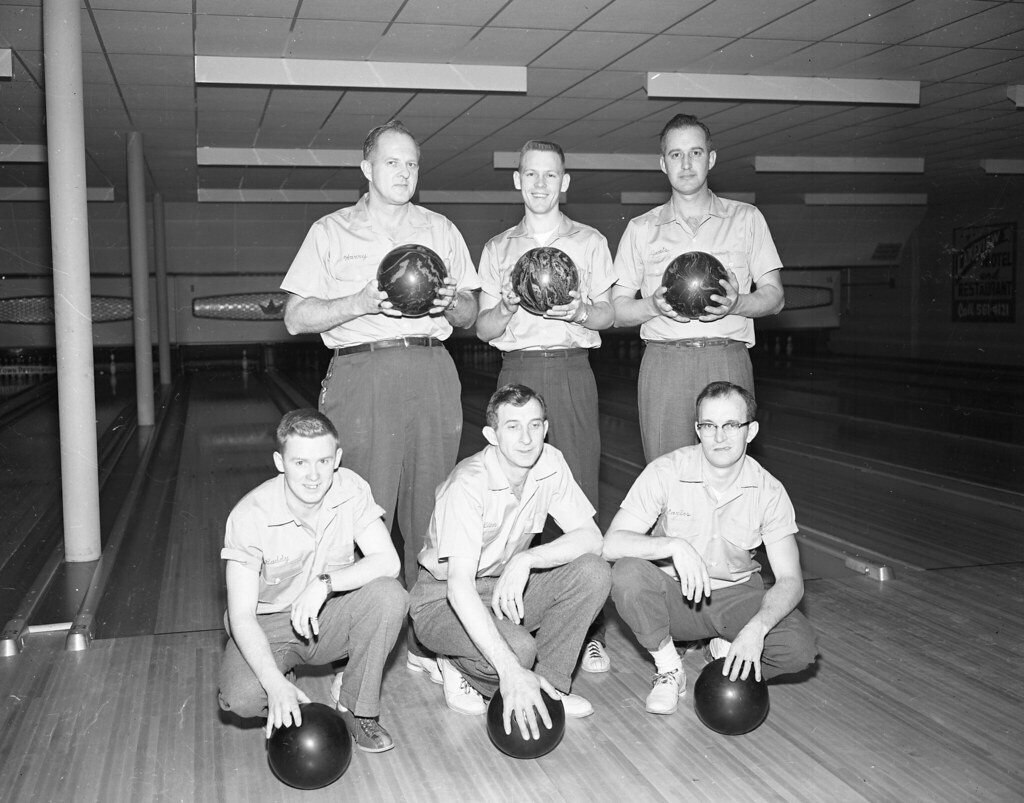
[316,574,332,594]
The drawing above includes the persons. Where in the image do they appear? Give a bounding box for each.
[604,382,817,716]
[410,384,612,740]
[216,408,409,754]
[614,115,785,662]
[476,142,618,672]
[277,124,481,686]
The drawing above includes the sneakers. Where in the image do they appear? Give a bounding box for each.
[645,667,688,715]
[710,638,734,660]
[407,650,443,684]
[556,688,594,718]
[437,651,486,715]
[335,703,395,752]
[580,639,610,673]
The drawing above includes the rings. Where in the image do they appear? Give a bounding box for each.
[745,661,751,662]
[566,311,567,315]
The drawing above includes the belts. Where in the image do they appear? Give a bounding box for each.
[338,338,442,355]
[651,339,737,346]
[505,348,586,358]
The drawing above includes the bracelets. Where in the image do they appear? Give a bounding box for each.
[449,300,458,311]
[577,306,589,323]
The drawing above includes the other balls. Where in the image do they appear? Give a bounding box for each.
[661,251,729,319]
[694,658,769,735]
[487,687,566,760]
[377,244,447,317]
[269,704,352,790]
[512,247,579,316]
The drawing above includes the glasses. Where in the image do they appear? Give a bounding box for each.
[696,420,751,433]
